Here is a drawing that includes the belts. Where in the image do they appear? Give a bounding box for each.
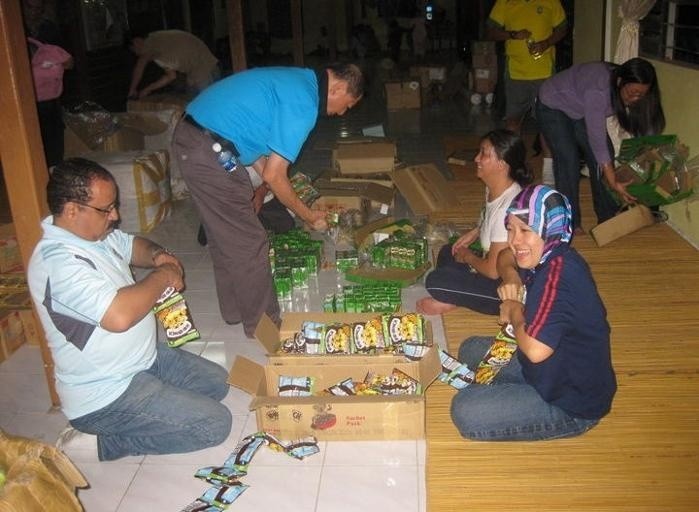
[182,112,239,158]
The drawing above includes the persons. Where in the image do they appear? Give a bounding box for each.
[416,127,534,315]
[124,29,224,100]
[171,63,364,340]
[451,185,618,442]
[26,157,232,462]
[21,0,68,166]
[481,0,570,186]
[536,58,665,236]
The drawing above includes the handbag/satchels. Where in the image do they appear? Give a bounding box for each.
[27,37,70,101]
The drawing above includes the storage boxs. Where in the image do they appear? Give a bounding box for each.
[254,311,433,362]
[227,344,442,441]
[58,89,192,168]
[0,221,42,366]
[267,134,462,311]
[377,40,500,112]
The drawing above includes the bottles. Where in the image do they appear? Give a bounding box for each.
[525,34,542,62]
[212,143,237,173]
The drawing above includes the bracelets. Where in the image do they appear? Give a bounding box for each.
[511,31,516,39]
[151,249,174,264]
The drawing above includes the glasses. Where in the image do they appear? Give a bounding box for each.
[68,200,116,218]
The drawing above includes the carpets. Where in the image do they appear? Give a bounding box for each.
[0,129,456,512]
